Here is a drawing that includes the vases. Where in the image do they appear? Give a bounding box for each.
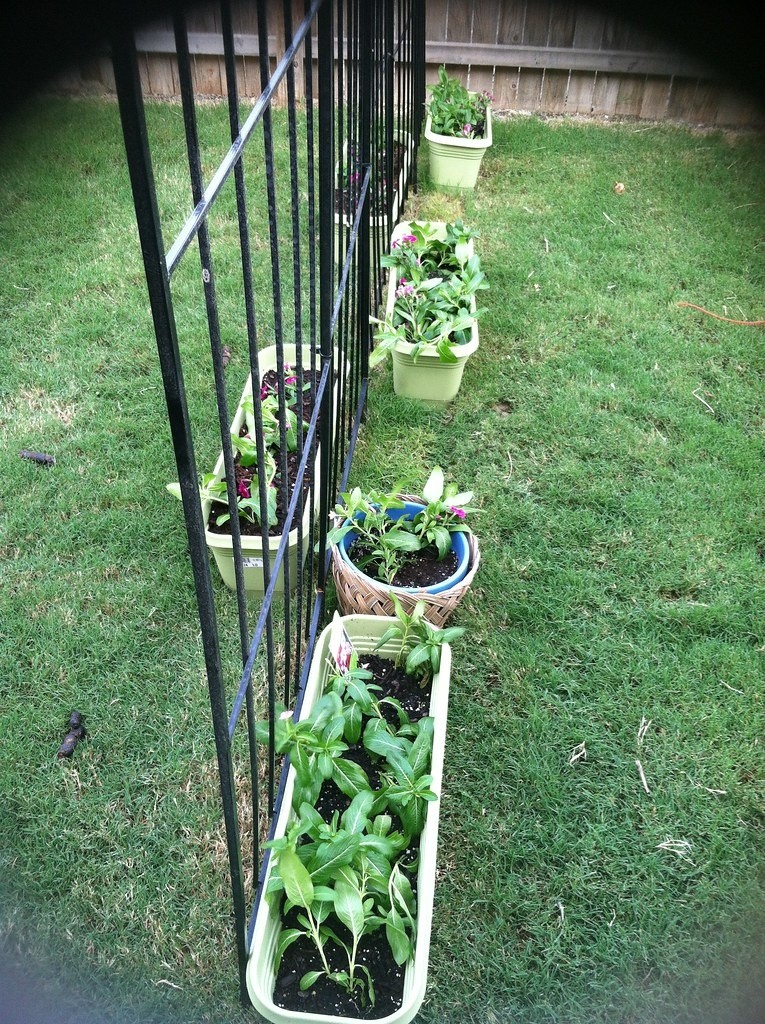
[425,91,492,192]
[386,221,478,400]
[202,342,350,592]
[332,494,483,629]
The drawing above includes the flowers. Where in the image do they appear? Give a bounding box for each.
[311,466,487,584]
[422,65,494,138]
[166,364,311,528]
[367,220,488,368]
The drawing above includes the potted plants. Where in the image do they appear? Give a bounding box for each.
[259,596,452,1024]
